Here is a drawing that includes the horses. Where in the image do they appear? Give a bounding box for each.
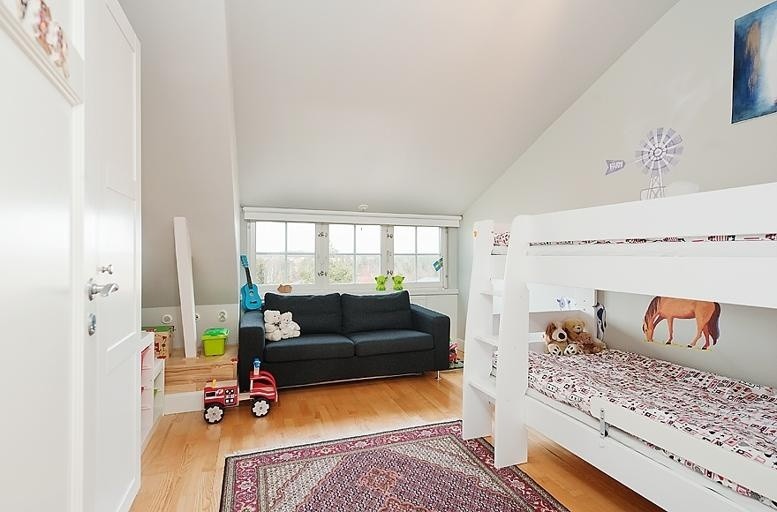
[641,296,721,351]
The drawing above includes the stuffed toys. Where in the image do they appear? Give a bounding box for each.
[375,275,388,291]
[391,275,405,290]
[540,319,608,357]
[263,310,284,342]
[277,284,292,294]
[278,311,301,340]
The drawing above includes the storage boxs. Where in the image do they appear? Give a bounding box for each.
[201,328,229,356]
[146,326,173,359]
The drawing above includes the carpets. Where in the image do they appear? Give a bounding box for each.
[218,417,571,510]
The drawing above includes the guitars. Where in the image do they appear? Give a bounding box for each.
[239,255,262,310]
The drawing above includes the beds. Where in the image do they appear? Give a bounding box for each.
[470,178,777,512]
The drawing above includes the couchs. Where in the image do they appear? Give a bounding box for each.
[239,293,450,394]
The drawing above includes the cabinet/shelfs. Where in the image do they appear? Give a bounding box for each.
[2,0,142,512]
[141,332,165,453]
